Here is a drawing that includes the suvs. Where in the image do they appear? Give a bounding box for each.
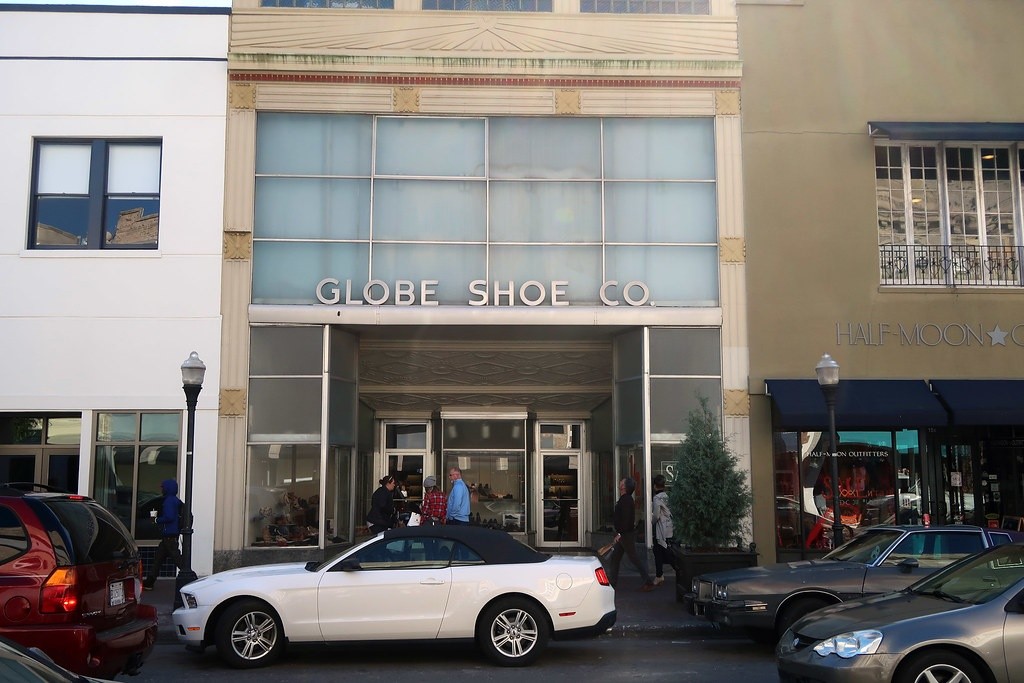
[0,482,158,680]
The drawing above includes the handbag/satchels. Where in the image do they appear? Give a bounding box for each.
[419,518,436,543]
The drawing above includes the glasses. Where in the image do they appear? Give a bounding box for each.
[449,474,459,476]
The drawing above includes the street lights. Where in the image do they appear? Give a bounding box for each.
[174,352,207,609]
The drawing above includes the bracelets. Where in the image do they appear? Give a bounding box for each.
[618,533,620,536]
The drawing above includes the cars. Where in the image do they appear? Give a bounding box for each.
[171,522,617,669]
[682,525,1020,652]
[481,500,561,526]
[775,537,1024,683]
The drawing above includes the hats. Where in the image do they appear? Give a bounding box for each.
[424,476,436,487]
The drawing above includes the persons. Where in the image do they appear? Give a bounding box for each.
[421,477,447,526]
[366,476,408,535]
[446,467,471,526]
[651,475,681,586]
[142,479,193,590]
[609,478,656,592]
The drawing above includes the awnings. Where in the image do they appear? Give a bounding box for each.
[764,378,1024,431]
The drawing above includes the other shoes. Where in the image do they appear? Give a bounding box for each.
[478,483,513,499]
[640,581,655,592]
[142,580,153,590]
[652,574,664,586]
[470,512,525,532]
